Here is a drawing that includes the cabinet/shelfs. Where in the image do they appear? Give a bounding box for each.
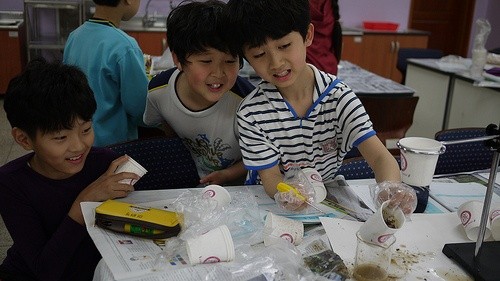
[125,30,168,57]
[0,19,27,95]
[339,36,428,84]
[23,0,88,63]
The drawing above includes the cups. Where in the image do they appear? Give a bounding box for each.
[185,224,236,264]
[264,211,305,247]
[202,184,231,208]
[359,200,406,244]
[115,155,149,188]
[457,199,491,242]
[297,167,327,204]
[488,198,500,242]
[398,136,442,188]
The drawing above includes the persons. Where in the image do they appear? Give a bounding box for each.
[224,0,416,215]
[0,58,165,281]
[63,0,148,149]
[143,0,256,186]
[306,0,342,75]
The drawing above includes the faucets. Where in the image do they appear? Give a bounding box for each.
[143,0,176,27]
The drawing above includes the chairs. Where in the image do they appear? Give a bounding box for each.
[95,47,500,191]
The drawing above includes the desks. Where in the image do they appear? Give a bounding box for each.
[401,59,500,146]
[236,58,415,155]
[81,169,500,281]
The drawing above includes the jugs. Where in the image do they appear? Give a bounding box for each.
[354,230,396,281]
